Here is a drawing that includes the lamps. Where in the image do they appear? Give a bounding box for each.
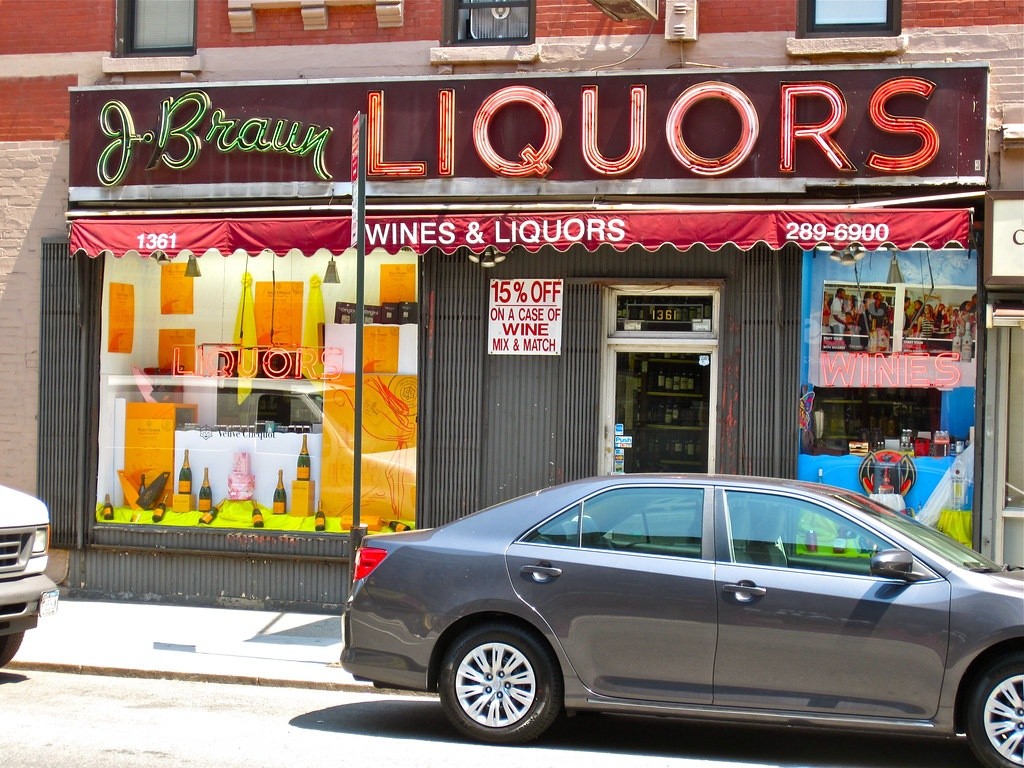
[492,245,505,262]
[480,249,495,267]
[852,245,866,259]
[885,250,904,284]
[183,252,202,277]
[840,246,857,265]
[829,249,842,261]
[149,251,156,260]
[323,254,341,283]
[467,252,480,262]
[156,251,170,264]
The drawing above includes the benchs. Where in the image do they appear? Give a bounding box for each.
[534,515,615,550]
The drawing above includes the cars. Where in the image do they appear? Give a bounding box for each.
[340,472,1024,768]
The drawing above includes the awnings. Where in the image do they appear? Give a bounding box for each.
[64,202,980,261]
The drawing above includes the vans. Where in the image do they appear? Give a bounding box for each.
[98,376,417,520]
[0,485,59,667]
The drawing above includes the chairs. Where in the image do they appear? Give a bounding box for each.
[733,527,754,563]
[750,512,788,567]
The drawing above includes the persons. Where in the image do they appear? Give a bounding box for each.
[821,287,977,359]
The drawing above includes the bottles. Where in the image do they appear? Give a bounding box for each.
[252,500,264,528]
[654,437,703,458]
[272,469,287,514]
[827,404,931,456]
[816,469,824,484]
[314,501,325,531]
[878,467,894,494]
[651,400,700,425]
[198,467,212,512]
[151,492,169,522]
[138,474,146,497]
[867,317,891,353]
[199,498,225,525]
[650,367,702,393]
[381,518,411,532]
[933,426,950,456]
[616,296,711,321]
[297,435,310,481]
[178,449,192,494]
[104,494,114,520]
[951,441,969,510]
[952,322,972,362]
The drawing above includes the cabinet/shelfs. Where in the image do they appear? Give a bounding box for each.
[616,293,712,475]
